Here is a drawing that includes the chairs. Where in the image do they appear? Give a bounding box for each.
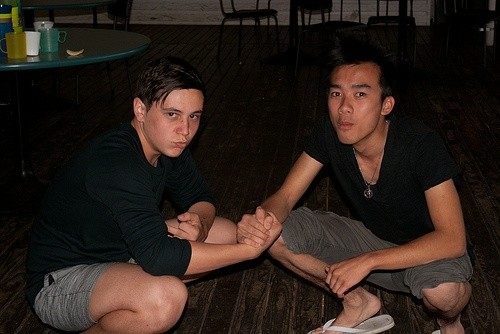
[216,0,500,81]
[55,0,133,104]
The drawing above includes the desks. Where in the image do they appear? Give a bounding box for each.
[0,0,152,179]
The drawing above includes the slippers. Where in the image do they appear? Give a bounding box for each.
[307,314,394,334]
[432,330,440,334]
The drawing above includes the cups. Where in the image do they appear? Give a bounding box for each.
[0,0,22,56]
[24,31,41,56]
[33,21,54,32]
[0,32,27,59]
[38,28,67,53]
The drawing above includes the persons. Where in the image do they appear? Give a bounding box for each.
[24,57,283,334]
[236,45,473,334]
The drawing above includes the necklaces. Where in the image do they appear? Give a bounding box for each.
[353,119,389,198]
